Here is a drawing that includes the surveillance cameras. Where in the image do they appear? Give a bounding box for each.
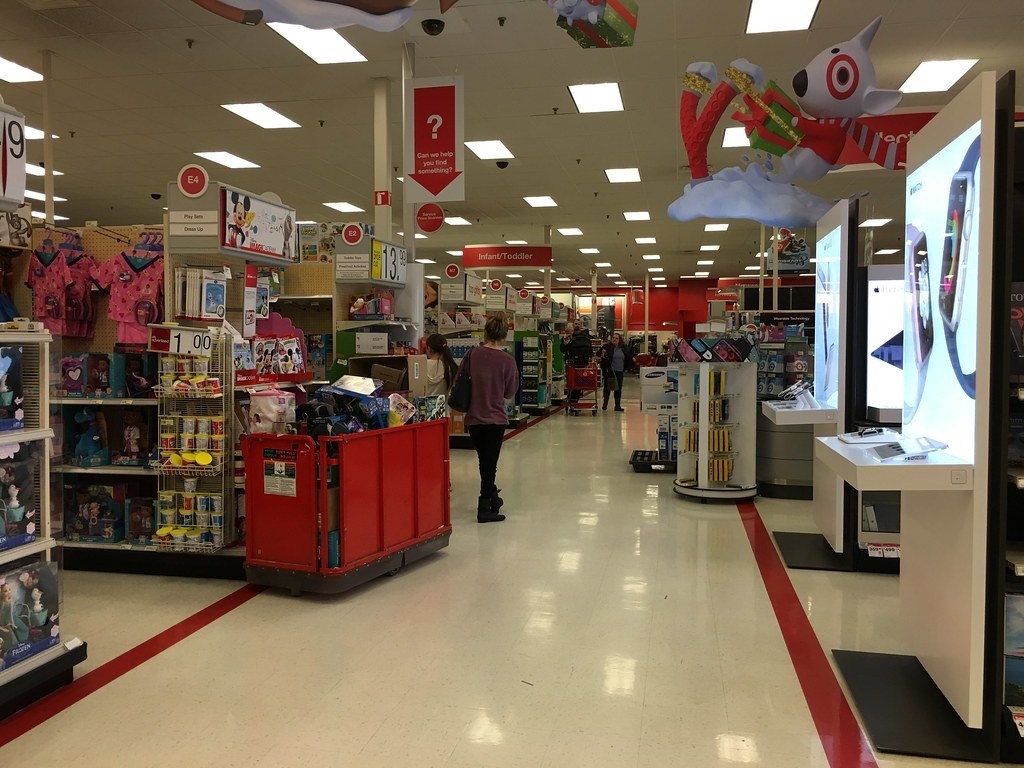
[151,194,162,200]
[496,162,509,169]
[575,279,580,282]
[421,19,444,36]
[39,162,45,169]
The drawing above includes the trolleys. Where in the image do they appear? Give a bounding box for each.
[635,353,666,377]
[565,363,599,417]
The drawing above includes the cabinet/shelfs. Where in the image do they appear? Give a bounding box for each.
[16,222,288,583]
[447,331,604,449]
[757,336,814,406]
[274,283,425,386]
[673,362,758,504]
[0,243,87,722]
[1005,374,1024,577]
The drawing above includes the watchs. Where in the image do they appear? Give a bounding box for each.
[938,135,981,400]
[905,225,932,424]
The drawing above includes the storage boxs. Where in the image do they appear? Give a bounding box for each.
[745,80,805,157]
[348,354,426,398]
[556,0,639,49]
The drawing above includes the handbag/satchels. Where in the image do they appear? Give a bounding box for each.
[447,349,473,414]
[606,377,620,391]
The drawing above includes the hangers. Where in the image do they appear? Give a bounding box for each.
[35,226,84,259]
[123,231,164,259]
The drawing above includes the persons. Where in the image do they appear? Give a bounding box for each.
[422,333,459,492]
[598,297,615,336]
[124,411,140,457]
[597,333,631,412]
[132,506,154,532]
[560,319,592,417]
[456,311,519,523]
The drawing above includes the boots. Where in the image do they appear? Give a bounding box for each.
[489,484,504,515]
[477,496,506,523]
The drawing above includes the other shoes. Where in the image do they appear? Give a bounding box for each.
[614,405,624,411]
[602,403,608,410]
